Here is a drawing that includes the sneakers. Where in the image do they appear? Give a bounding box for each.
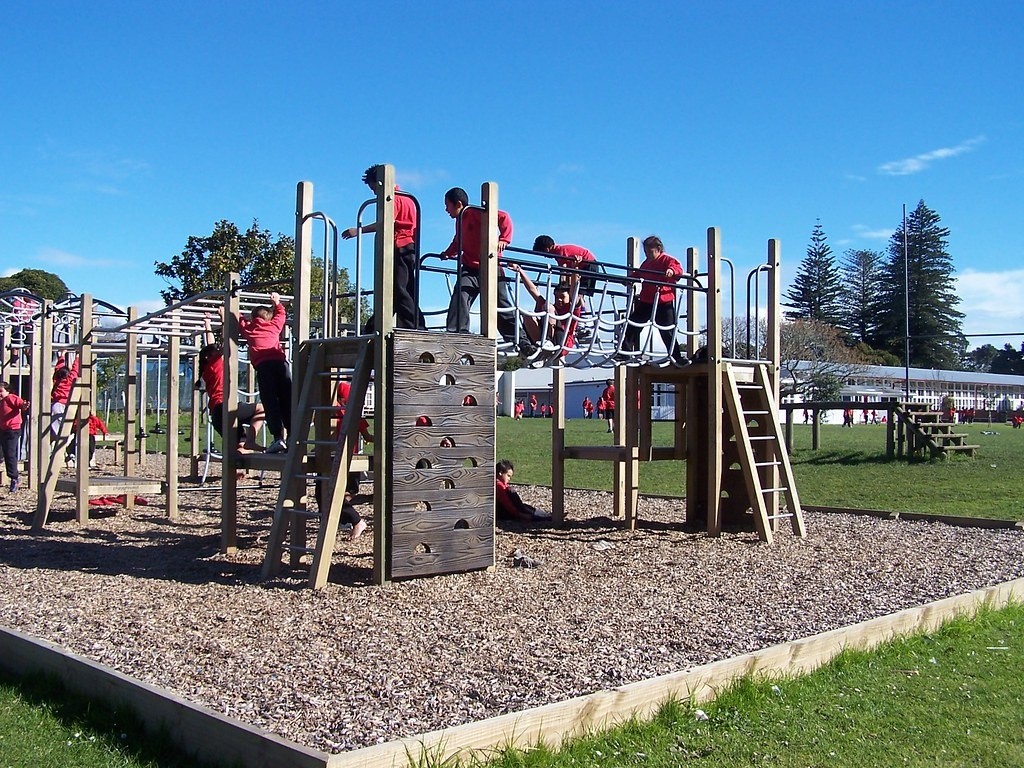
[499,550,521,561]
[591,539,616,551]
[512,555,543,568]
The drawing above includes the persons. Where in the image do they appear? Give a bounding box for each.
[194,305,268,454]
[341,163,428,335]
[602,379,615,433]
[842,409,886,428]
[439,188,530,346]
[496,460,568,522]
[611,236,682,361]
[949,406,976,425]
[582,396,606,419]
[531,235,599,338]
[10,295,36,367]
[496,392,503,418]
[514,394,553,420]
[1012,415,1022,429]
[315,383,373,540]
[0,382,31,494]
[50,350,79,444]
[803,409,809,424]
[65,409,110,468]
[507,262,585,357]
[239,292,292,453]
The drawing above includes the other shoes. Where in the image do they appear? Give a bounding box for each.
[89,466,94,470]
[613,352,642,363]
[262,439,288,455]
[533,356,567,367]
[9,478,22,493]
[25,363,30,368]
[336,520,353,542]
[669,355,688,367]
[606,428,614,433]
[65,454,75,463]
[11,361,16,366]
[517,345,536,360]
